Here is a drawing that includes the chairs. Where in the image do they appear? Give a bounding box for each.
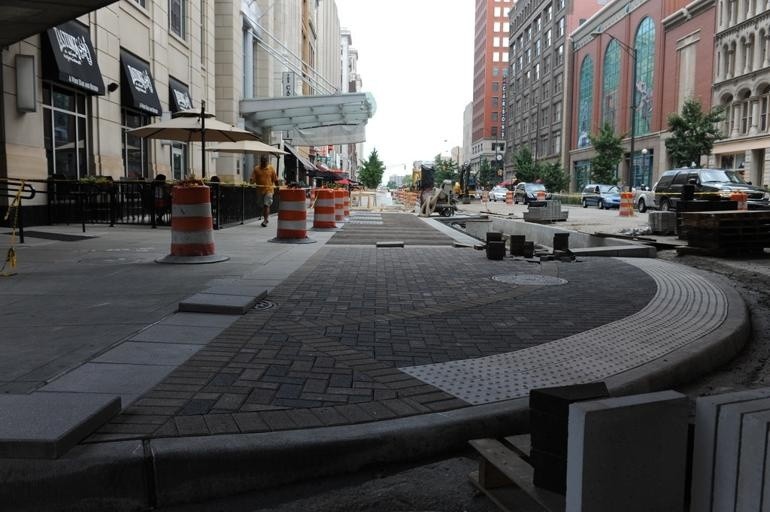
[52,174,280,230]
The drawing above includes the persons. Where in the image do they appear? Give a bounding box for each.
[249,153,280,229]
[209,175,222,217]
[150,175,172,224]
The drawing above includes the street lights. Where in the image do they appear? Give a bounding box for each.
[591,31,637,192]
[510,91,539,165]
[641,149,648,184]
[444,140,459,181]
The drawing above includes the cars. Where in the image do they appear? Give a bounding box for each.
[514,182,552,205]
[489,187,509,202]
[581,168,770,212]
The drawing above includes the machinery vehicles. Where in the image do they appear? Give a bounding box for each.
[453,159,479,204]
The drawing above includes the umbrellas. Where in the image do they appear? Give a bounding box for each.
[124,99,262,180]
[200,136,293,155]
